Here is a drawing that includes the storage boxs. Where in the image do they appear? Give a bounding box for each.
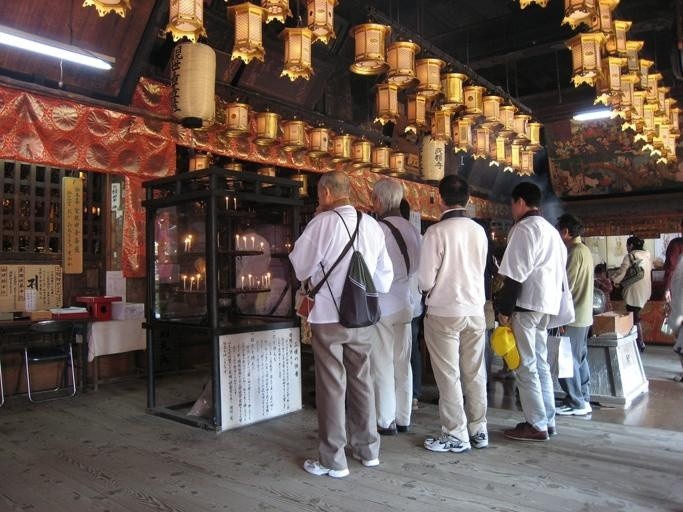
[112,301,144,321]
[51,308,90,321]
[592,309,636,340]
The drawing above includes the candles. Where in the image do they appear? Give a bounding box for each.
[225,196,229,210]
[182,233,274,294]
[233,198,237,210]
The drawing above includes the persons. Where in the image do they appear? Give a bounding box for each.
[668,253,682,383]
[492,245,515,378]
[400,198,424,409]
[423,174,490,453]
[287,171,396,479]
[555,215,595,416]
[663,222,683,310]
[595,264,614,315]
[613,236,653,352]
[372,178,423,434]
[497,181,569,441]
[430,218,495,405]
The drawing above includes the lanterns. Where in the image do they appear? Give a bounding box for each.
[306,0,338,44]
[262,0,294,24]
[187,151,211,182]
[227,3,268,65]
[349,24,544,181]
[561,0,682,166]
[165,0,208,44]
[226,103,405,177]
[279,26,315,82]
[169,41,215,129]
[82,0,132,18]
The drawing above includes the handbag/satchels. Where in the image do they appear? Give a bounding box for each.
[294,286,317,318]
[546,323,575,380]
[619,252,644,286]
[483,299,498,330]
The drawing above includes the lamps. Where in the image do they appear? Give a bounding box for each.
[573,105,612,122]
[0,25,116,73]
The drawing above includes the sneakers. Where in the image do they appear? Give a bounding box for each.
[505,419,558,442]
[555,397,593,416]
[409,399,419,411]
[353,449,381,467]
[425,429,490,454]
[303,458,350,479]
[376,420,412,435]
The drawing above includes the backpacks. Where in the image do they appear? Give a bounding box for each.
[338,249,381,330]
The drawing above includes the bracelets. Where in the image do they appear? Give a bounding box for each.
[665,288,670,291]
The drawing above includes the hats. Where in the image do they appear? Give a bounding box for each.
[488,326,522,371]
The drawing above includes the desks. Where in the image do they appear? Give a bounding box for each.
[87,317,150,391]
[0,315,89,399]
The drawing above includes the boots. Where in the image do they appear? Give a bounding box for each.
[634,323,645,353]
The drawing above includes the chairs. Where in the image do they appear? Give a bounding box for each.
[14,320,78,402]
